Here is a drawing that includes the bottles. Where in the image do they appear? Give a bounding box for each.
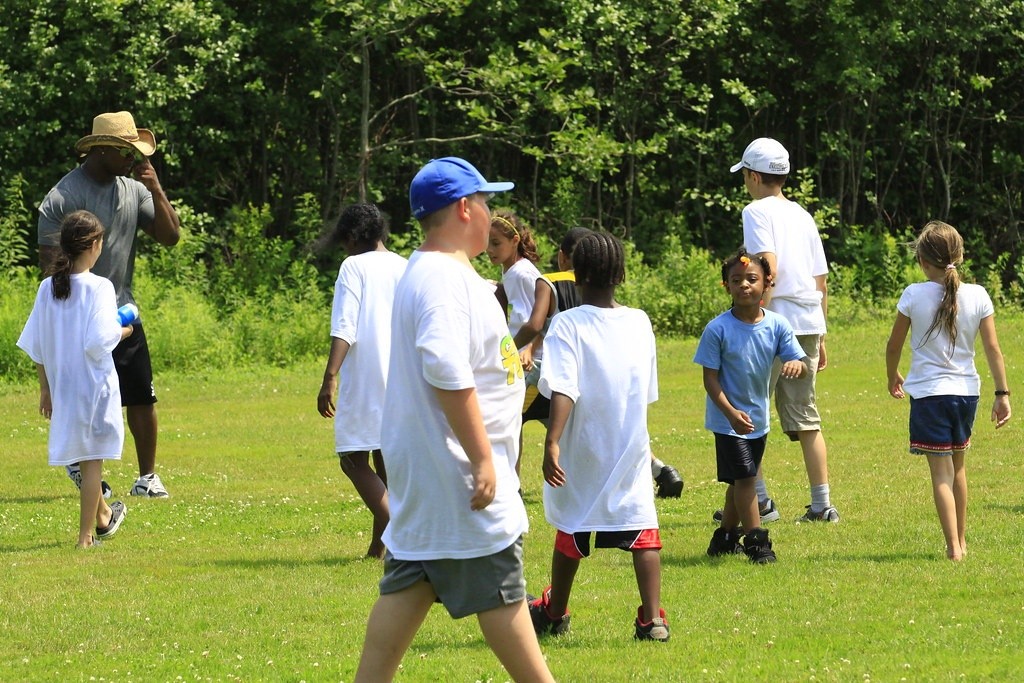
[117,302,139,326]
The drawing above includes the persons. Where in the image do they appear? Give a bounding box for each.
[526,232,671,643]
[353,154,559,683]
[317,203,409,557]
[15,207,132,551]
[34,111,180,501]
[728,138,841,523]
[484,209,687,502]
[885,219,1013,564]
[690,246,808,566]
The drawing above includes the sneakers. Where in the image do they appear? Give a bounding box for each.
[524,584,572,634]
[95,501,127,538]
[654,464,684,498]
[130,473,169,500]
[66,470,111,499]
[632,602,670,643]
[796,504,841,523]
[706,527,744,558]
[736,529,776,564]
[713,497,781,525]
[74,535,102,548]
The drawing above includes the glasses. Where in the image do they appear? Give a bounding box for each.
[112,145,143,161]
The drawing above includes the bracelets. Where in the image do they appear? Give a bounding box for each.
[994,389,1010,397]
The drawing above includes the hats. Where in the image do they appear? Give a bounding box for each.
[73,110,157,156]
[729,137,791,174]
[410,155,516,220]
